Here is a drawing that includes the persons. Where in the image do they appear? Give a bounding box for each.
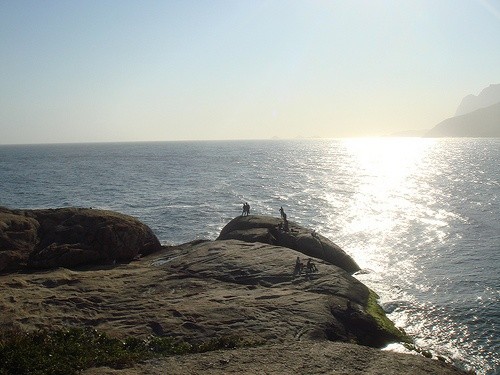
[293,256,302,275]
[307,258,318,272]
[278,207,289,233]
[242,202,251,217]
[313,230,318,237]
